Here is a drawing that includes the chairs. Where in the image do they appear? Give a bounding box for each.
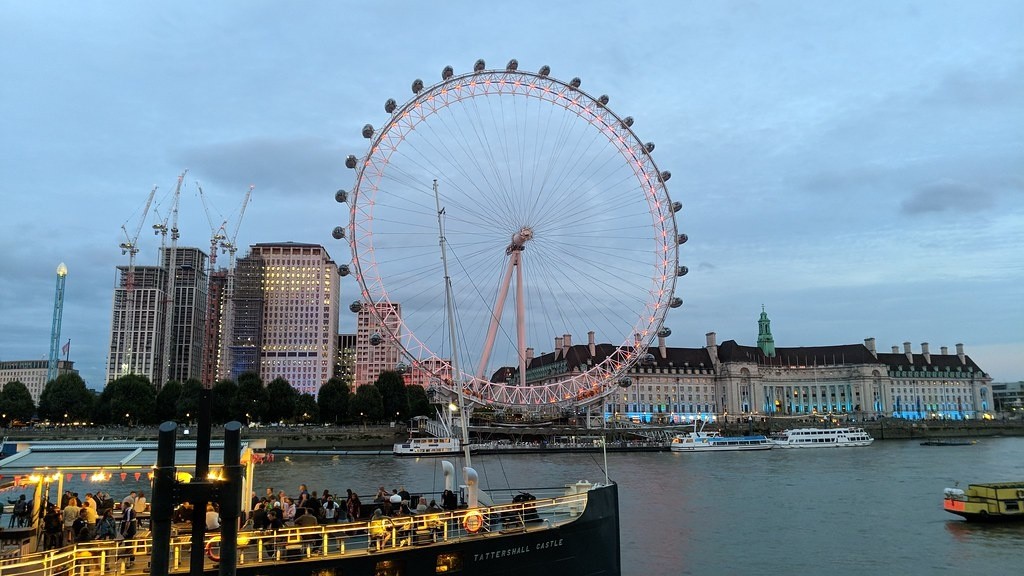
[0,491,457,576]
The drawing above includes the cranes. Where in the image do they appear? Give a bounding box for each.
[117,167,257,391]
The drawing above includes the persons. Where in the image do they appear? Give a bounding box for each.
[171,494,222,535]
[696,433,701,438]
[674,432,691,438]
[0,485,149,550]
[368,483,458,553]
[237,484,361,564]
[719,433,722,437]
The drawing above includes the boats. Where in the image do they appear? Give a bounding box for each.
[0,178,623,576]
[393,436,479,458]
[669,431,777,452]
[774,426,874,449]
[943,479,1024,524]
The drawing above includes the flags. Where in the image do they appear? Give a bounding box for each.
[719,429,721,431]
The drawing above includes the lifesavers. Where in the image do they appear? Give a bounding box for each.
[462,512,482,533]
[206,537,222,561]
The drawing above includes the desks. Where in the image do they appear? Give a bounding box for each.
[440,504,468,512]
[96,514,150,532]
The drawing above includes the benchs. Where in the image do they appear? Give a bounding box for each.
[0,549,20,566]
[133,530,151,556]
